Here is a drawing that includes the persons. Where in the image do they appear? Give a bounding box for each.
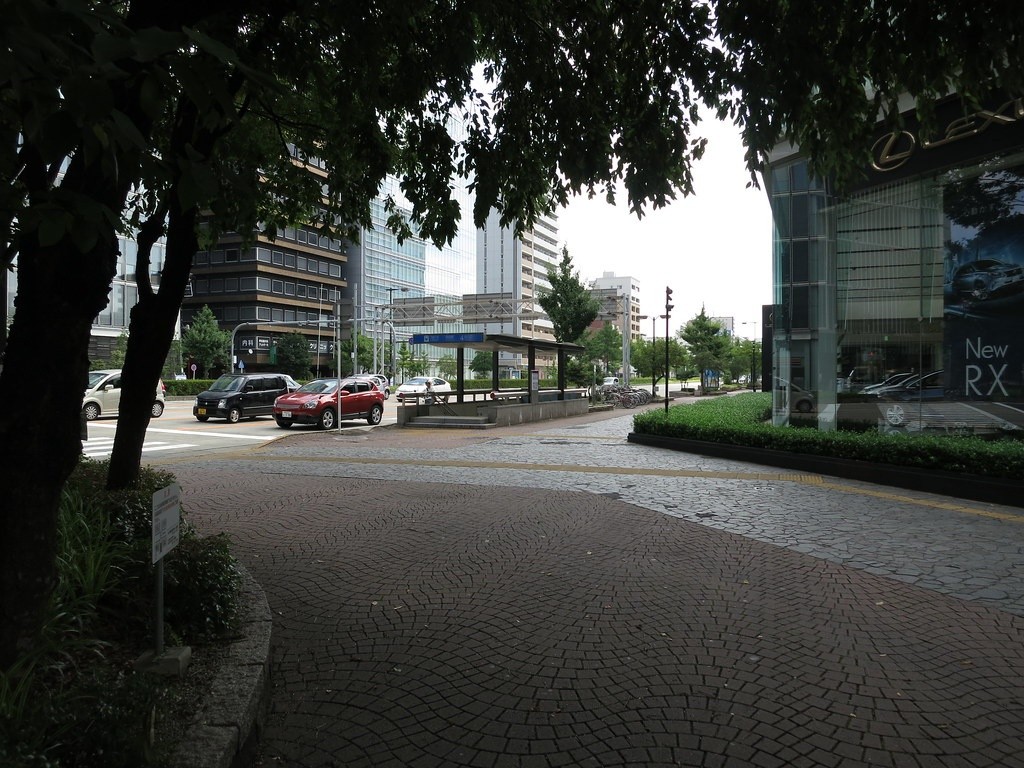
[422,379,437,405]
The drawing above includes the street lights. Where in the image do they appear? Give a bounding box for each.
[388,286,411,373]
[640,332,647,339]
[742,320,756,392]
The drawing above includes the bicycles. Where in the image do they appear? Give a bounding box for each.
[596,383,652,409]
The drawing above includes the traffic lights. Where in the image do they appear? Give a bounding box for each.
[661,286,673,319]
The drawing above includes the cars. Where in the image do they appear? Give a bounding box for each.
[395,377,451,403]
[373,374,390,400]
[369,377,383,391]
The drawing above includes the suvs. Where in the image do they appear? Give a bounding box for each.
[272,378,384,430]
[859,369,990,427]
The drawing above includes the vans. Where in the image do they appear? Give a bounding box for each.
[82,369,167,421]
[193,373,302,424]
